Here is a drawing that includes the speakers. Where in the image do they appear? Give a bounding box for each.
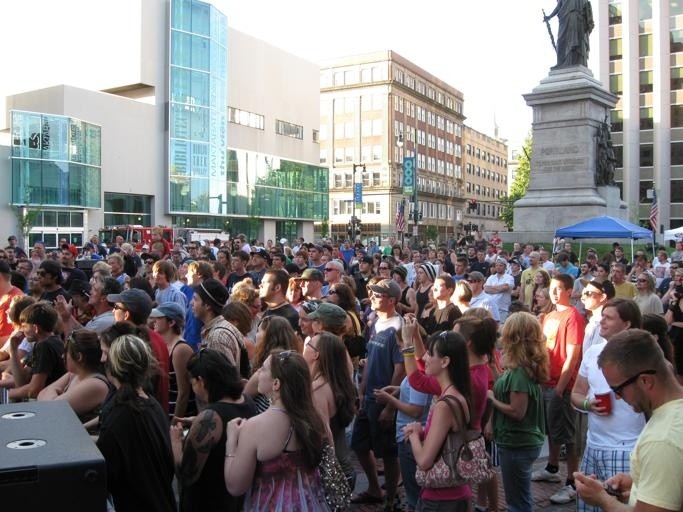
[0,399,107,512]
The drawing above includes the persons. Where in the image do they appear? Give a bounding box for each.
[540,0,594,67]
[0,225,683,512]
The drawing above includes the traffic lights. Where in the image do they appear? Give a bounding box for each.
[471,198,476,209]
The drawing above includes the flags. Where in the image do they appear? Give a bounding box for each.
[650,186,658,229]
[396,202,405,232]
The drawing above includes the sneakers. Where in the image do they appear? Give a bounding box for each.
[549,484,576,504]
[530,464,561,483]
[376,457,384,472]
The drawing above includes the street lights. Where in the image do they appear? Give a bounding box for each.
[352,163,366,239]
[396,130,423,246]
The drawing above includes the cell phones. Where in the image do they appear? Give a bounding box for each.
[582,475,620,497]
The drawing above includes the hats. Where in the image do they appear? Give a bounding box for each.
[142,244,148,250]
[61,242,78,258]
[194,278,229,311]
[463,271,483,281]
[250,246,269,260]
[509,256,520,264]
[496,256,508,270]
[148,302,186,325]
[140,253,160,262]
[294,263,436,327]
[308,241,323,256]
[105,288,152,313]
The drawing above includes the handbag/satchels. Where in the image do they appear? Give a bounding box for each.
[319,438,351,511]
[415,394,494,488]
[475,439,506,512]
[344,312,366,357]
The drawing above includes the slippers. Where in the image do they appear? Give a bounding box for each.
[349,491,385,503]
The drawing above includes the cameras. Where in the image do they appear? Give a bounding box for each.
[181,423,190,440]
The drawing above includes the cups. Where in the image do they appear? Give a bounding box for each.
[594,389,612,414]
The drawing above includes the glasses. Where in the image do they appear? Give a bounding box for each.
[609,369,656,396]
[143,261,154,264]
[304,335,319,353]
[278,349,296,368]
[670,266,677,269]
[111,303,126,312]
[636,278,645,282]
[195,347,205,366]
[584,291,601,296]
[66,329,76,344]
[36,270,47,277]
[439,329,448,346]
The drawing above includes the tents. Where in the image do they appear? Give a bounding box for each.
[664,227,683,249]
[551,216,655,264]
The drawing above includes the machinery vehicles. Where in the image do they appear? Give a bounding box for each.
[94,222,230,258]
